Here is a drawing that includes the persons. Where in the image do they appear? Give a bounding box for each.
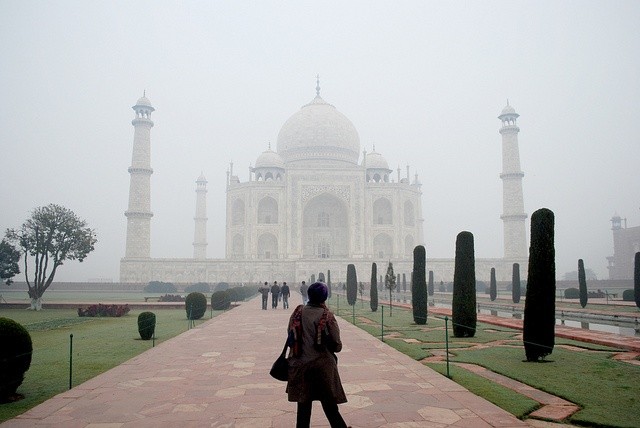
[284,282,352,428]
[280,281,291,309]
[270,281,280,308]
[258,281,270,310]
[299,280,309,305]
